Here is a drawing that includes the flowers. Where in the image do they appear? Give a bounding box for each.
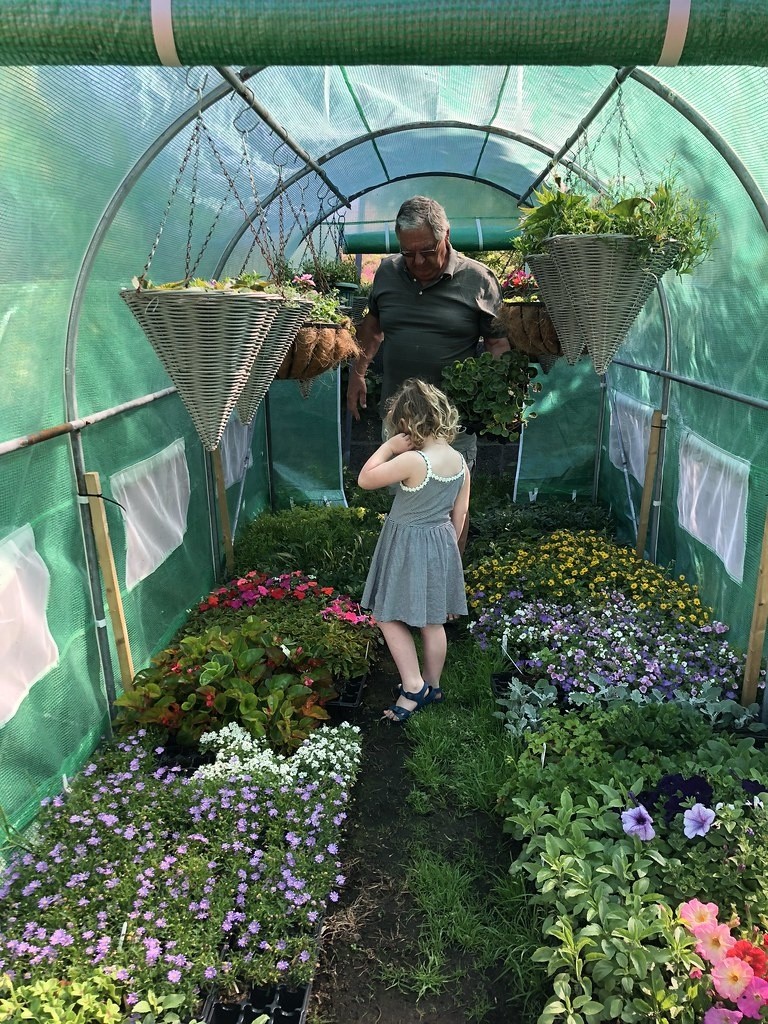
[500,270,540,286]
[614,769,765,871]
[147,274,336,321]
[674,899,768,1024]
[465,528,767,706]
[0,570,380,1024]
[502,294,539,302]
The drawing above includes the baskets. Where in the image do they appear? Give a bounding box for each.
[539,354,560,374]
[353,297,369,323]
[236,299,317,424]
[525,254,586,365]
[295,377,315,399]
[335,306,352,315]
[119,289,286,452]
[543,234,686,375]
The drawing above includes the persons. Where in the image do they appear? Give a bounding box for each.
[357,379,473,724]
[345,196,509,622]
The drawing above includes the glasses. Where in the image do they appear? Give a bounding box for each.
[400,241,440,257]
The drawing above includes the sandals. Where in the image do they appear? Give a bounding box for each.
[434,688,445,703]
[384,681,435,724]
